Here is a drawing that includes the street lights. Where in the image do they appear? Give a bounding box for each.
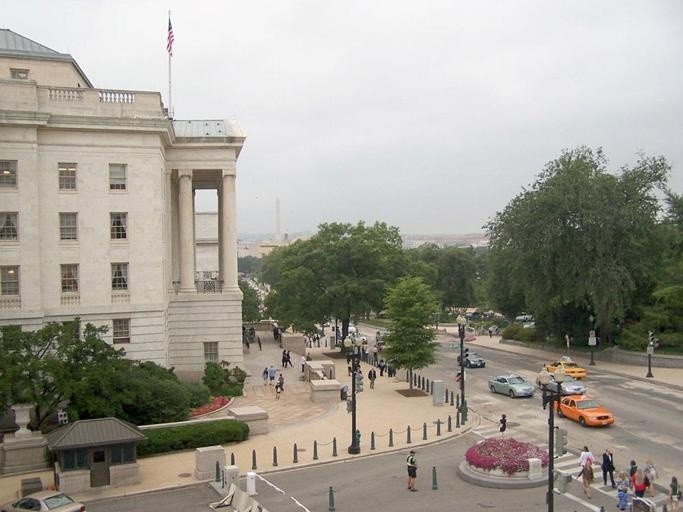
[536,360,572,512]
[342,335,363,456]
[454,313,469,412]
[585,315,598,366]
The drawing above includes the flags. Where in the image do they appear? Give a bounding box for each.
[165,17,175,58]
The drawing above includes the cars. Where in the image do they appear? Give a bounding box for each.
[537,367,586,397]
[357,331,368,344]
[487,373,536,400]
[540,354,587,381]
[455,350,487,368]
[514,311,533,323]
[554,392,617,429]
[319,320,330,328]
[346,323,356,333]
[0,490,86,512]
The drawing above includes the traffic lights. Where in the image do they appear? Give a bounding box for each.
[648,332,655,346]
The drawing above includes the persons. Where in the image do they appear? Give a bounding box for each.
[346,358,352,376]
[262,349,312,400]
[355,343,393,389]
[489,326,493,339]
[406,450,418,491]
[497,413,507,432]
[574,445,678,512]
